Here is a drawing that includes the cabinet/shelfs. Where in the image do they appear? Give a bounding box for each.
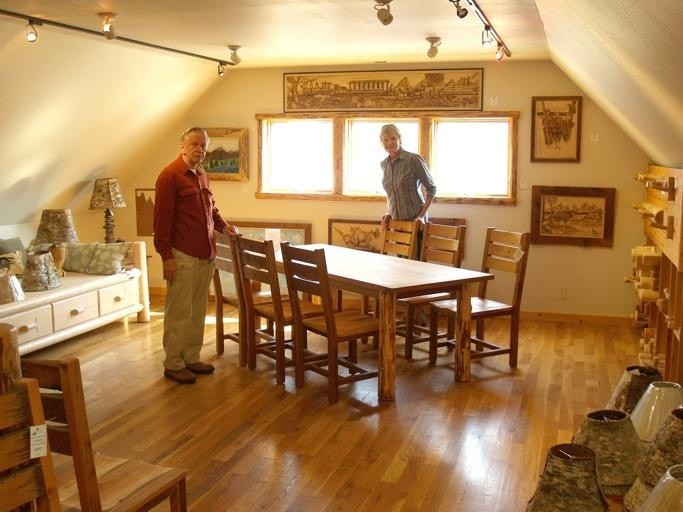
[631,164,683,386]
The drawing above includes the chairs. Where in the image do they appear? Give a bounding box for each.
[0,375,62,512]
[360,215,416,351]
[393,216,467,366]
[233,233,328,387]
[208,229,295,369]
[429,222,530,370]
[277,241,381,407]
[11,349,189,512]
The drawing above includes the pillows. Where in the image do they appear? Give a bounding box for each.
[86,240,133,276]
[0,237,28,267]
[60,240,99,276]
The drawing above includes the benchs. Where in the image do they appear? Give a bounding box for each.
[0,219,151,359]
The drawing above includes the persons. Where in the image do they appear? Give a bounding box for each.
[377,124,435,263]
[152,127,240,384]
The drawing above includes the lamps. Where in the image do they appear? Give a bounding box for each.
[21,14,44,43]
[223,44,244,66]
[86,176,130,245]
[446,0,511,62]
[423,35,442,59]
[96,12,122,40]
[34,208,80,279]
[371,0,395,26]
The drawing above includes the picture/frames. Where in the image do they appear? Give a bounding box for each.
[326,217,386,255]
[282,66,483,112]
[198,126,250,184]
[528,183,616,249]
[528,94,582,164]
[207,221,313,304]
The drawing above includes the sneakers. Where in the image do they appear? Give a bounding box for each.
[164,368,197,384]
[185,362,215,372]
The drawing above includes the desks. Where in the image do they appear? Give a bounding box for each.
[264,241,478,406]
